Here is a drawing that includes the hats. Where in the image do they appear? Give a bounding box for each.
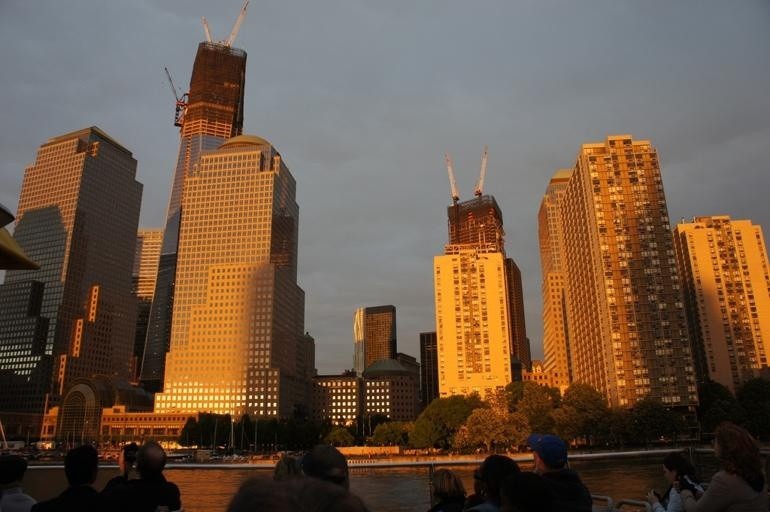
[299,440,348,482]
[530,433,567,462]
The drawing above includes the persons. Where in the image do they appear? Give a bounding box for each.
[1,414,769,512]
